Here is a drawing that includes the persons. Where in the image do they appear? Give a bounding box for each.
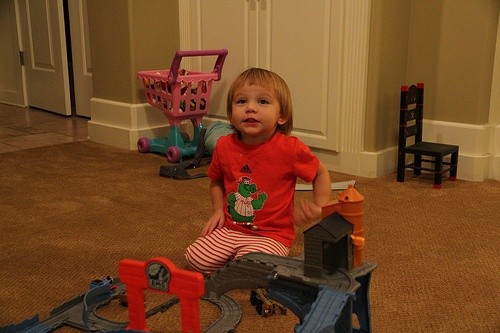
[184,68,331,273]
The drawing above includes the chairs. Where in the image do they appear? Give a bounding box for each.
[397,83,459,189]
